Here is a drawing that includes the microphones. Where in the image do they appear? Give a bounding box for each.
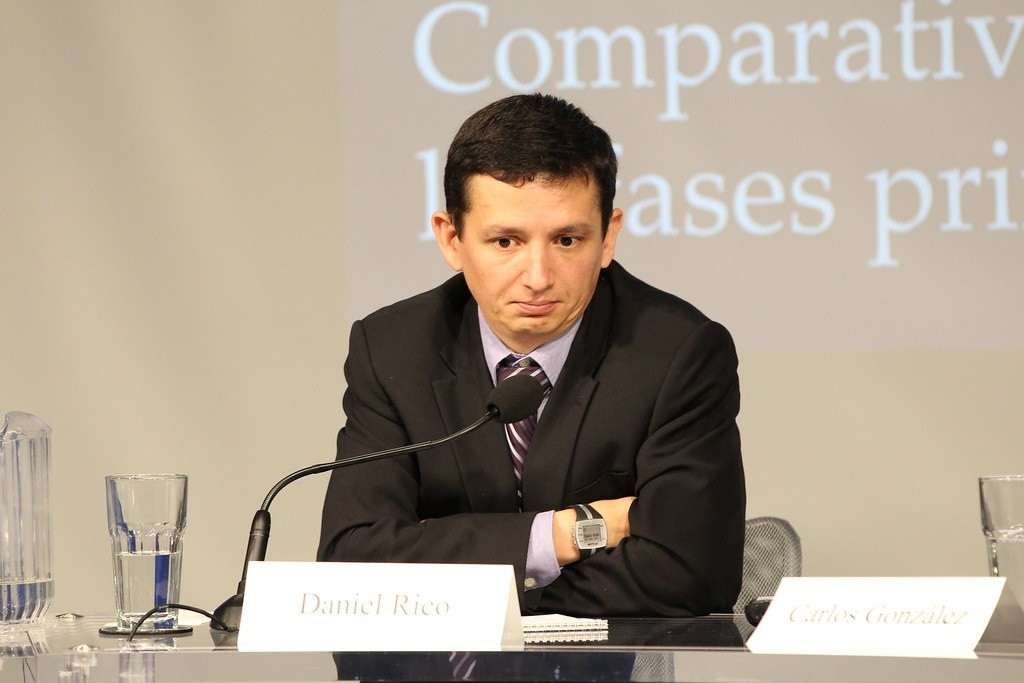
[211,373,546,630]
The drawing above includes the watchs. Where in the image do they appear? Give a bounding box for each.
[566,504,607,560]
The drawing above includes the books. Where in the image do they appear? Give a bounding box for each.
[523,632,610,643]
[522,614,609,631]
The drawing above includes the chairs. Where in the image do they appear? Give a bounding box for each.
[731,518,801,646]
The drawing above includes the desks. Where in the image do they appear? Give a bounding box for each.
[0,618,1024,683]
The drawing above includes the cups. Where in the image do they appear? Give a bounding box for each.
[103,473,189,628]
[0,410,53,656]
[978,475,1024,642]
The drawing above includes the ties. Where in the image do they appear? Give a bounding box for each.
[496,357,553,511]
[447,651,478,681]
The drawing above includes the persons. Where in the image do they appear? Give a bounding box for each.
[315,92,745,617]
[330,652,636,683]
